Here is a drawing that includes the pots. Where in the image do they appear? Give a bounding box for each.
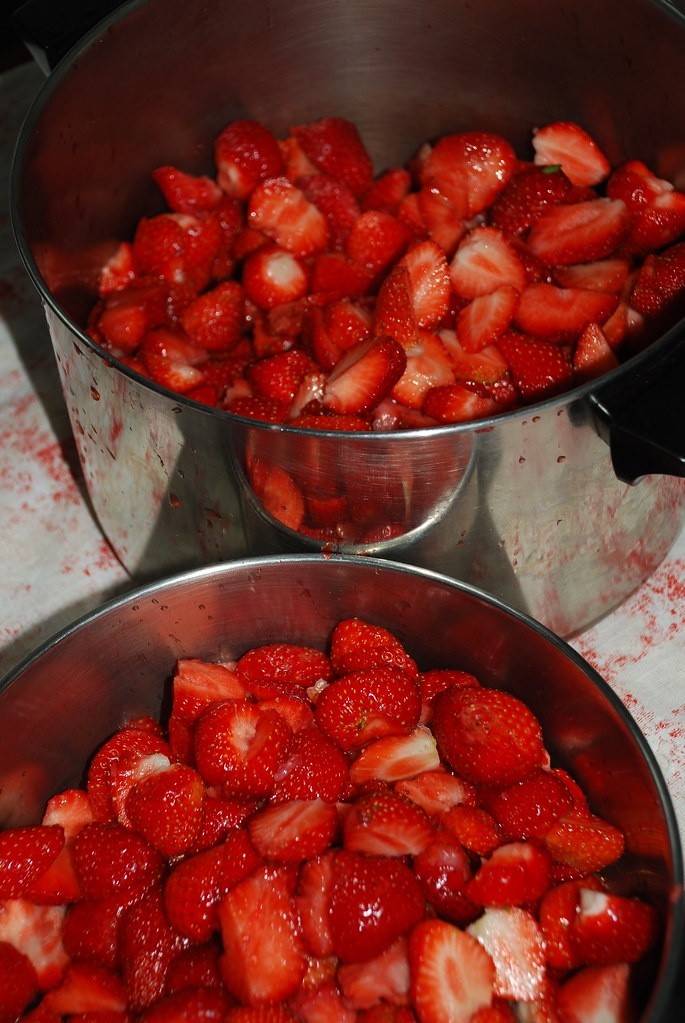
[0,0,685,648]
[0,556,685,1022]
[216,410,483,565]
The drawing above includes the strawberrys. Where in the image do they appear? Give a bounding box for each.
[79,117,685,434]
[0,617,653,1023]
